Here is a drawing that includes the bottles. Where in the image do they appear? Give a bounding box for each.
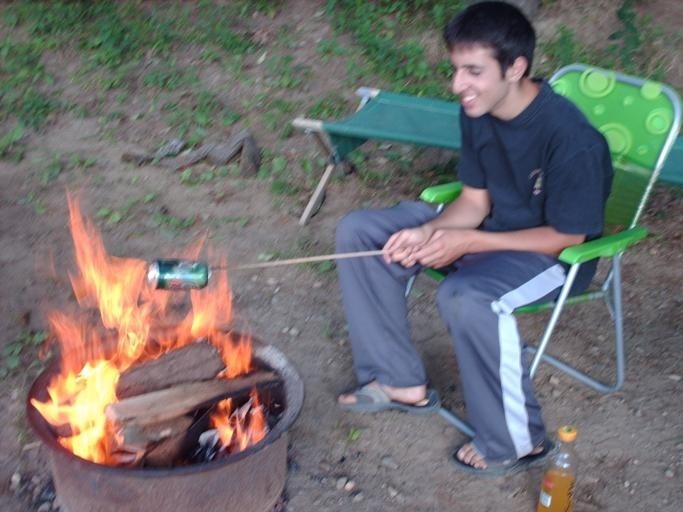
[534,424,579,512]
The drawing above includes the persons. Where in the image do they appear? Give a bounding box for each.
[335,1,614,476]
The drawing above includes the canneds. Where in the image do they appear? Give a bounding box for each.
[146,259,208,290]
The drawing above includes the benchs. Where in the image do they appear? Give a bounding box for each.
[292,87,683,227]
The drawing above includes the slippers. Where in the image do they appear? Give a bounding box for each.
[335,386,440,415]
[448,436,560,476]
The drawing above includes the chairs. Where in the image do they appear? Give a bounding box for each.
[402,63,683,440]
